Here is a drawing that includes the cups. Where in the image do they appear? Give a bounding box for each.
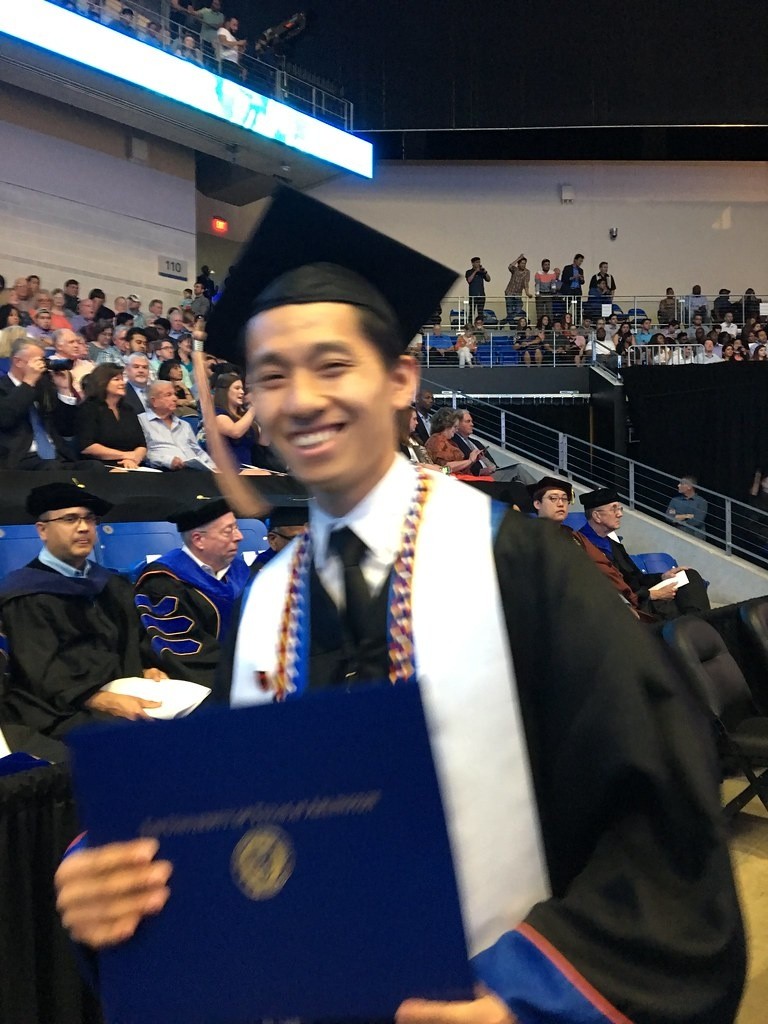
[440,465,450,476]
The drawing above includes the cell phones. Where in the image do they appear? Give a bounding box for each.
[480,446,490,454]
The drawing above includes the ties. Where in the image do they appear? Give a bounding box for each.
[327,525,383,680]
[466,438,494,468]
[28,402,55,460]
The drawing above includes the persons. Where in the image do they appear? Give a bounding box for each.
[250,504,309,578]
[714,289,731,323]
[0,266,302,476]
[590,262,616,300]
[44,0,247,84]
[133,495,250,692]
[0,482,171,747]
[739,288,762,323]
[403,312,768,367]
[465,257,491,322]
[504,253,532,324]
[578,488,711,621]
[535,259,557,323]
[666,475,708,541]
[588,279,612,314]
[560,254,584,313]
[685,285,709,324]
[657,288,681,329]
[54,181,748,1024]
[397,389,538,513]
[526,476,640,620]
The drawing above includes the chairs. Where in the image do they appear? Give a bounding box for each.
[0,510,768,843]
[423,305,647,367]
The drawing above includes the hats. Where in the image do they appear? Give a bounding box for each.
[263,503,309,528]
[26,482,114,519]
[127,294,141,303]
[527,475,575,504]
[192,179,462,516]
[579,488,622,509]
[167,494,233,531]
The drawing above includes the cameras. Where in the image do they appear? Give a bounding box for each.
[41,356,74,375]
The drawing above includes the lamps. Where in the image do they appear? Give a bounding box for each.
[609,228,618,239]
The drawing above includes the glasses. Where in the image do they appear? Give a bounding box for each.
[270,530,296,540]
[539,494,568,503]
[40,512,96,523]
